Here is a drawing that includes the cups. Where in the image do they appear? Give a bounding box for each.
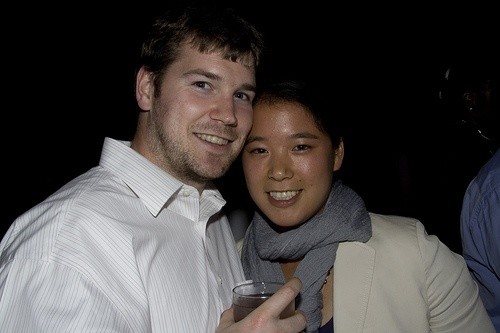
[232,281,296,323]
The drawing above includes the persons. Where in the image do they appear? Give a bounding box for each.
[234,79,498,332]
[459,147,500,332]
[0,5,308,332]
